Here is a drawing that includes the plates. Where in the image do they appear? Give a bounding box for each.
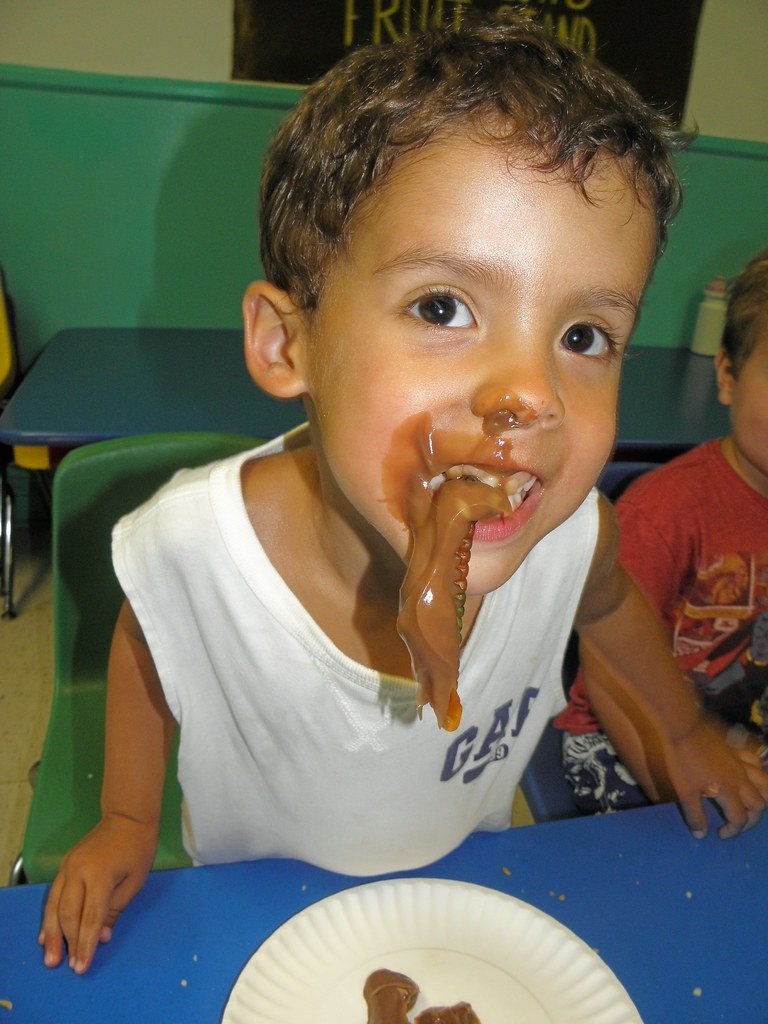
[221,876,648,1024]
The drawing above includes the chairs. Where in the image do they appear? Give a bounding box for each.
[518,459,662,822]
[6,432,270,879]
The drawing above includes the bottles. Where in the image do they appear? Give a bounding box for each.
[690,275,729,358]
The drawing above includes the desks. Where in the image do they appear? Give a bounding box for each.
[0,327,732,622]
[0,795,768,1024]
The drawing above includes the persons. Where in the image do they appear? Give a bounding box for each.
[35,3,768,976]
[549,248,768,815]
